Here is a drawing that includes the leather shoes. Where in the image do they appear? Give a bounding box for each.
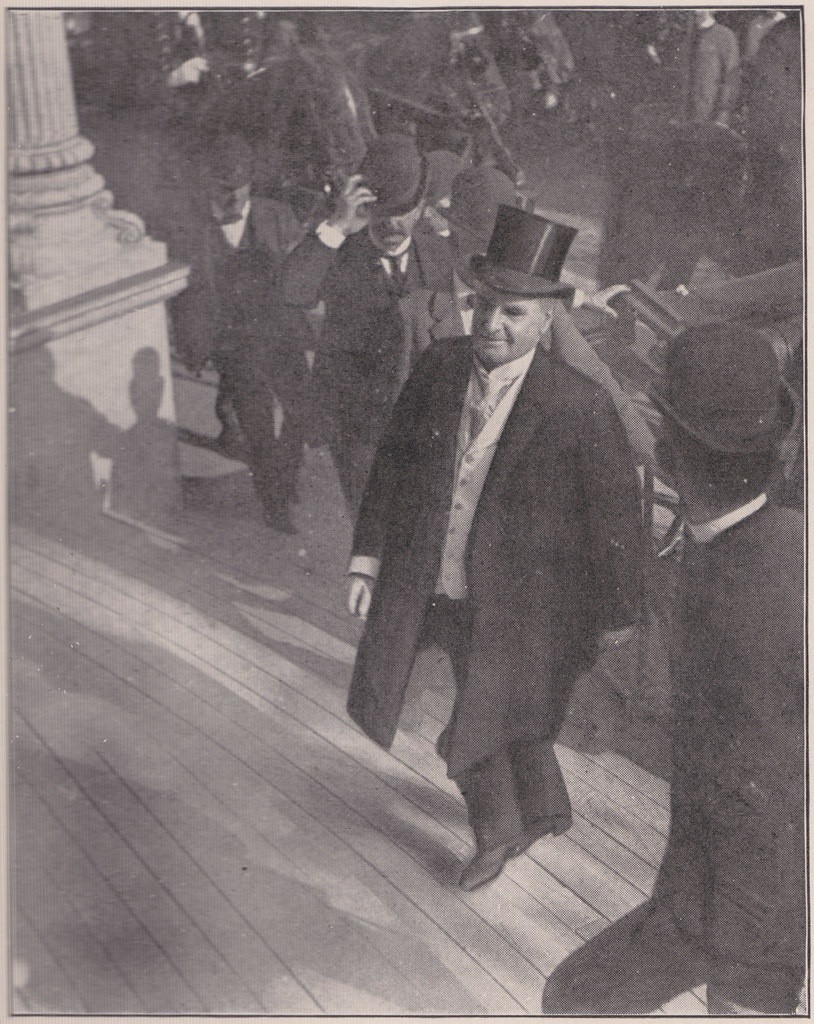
[517,812,573,854]
[458,834,520,892]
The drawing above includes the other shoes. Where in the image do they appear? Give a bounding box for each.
[267,513,295,536]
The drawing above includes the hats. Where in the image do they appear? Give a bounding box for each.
[207,145,253,190]
[645,322,802,455]
[436,166,517,246]
[428,149,466,201]
[357,134,428,219]
[470,202,578,297]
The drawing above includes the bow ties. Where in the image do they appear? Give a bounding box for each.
[221,213,243,224]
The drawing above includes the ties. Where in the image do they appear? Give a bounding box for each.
[466,365,517,439]
[373,244,409,287]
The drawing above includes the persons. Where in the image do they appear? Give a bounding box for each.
[280,133,466,526]
[169,127,318,534]
[65,12,803,295]
[538,326,805,1015]
[346,202,652,893]
[360,168,653,472]
